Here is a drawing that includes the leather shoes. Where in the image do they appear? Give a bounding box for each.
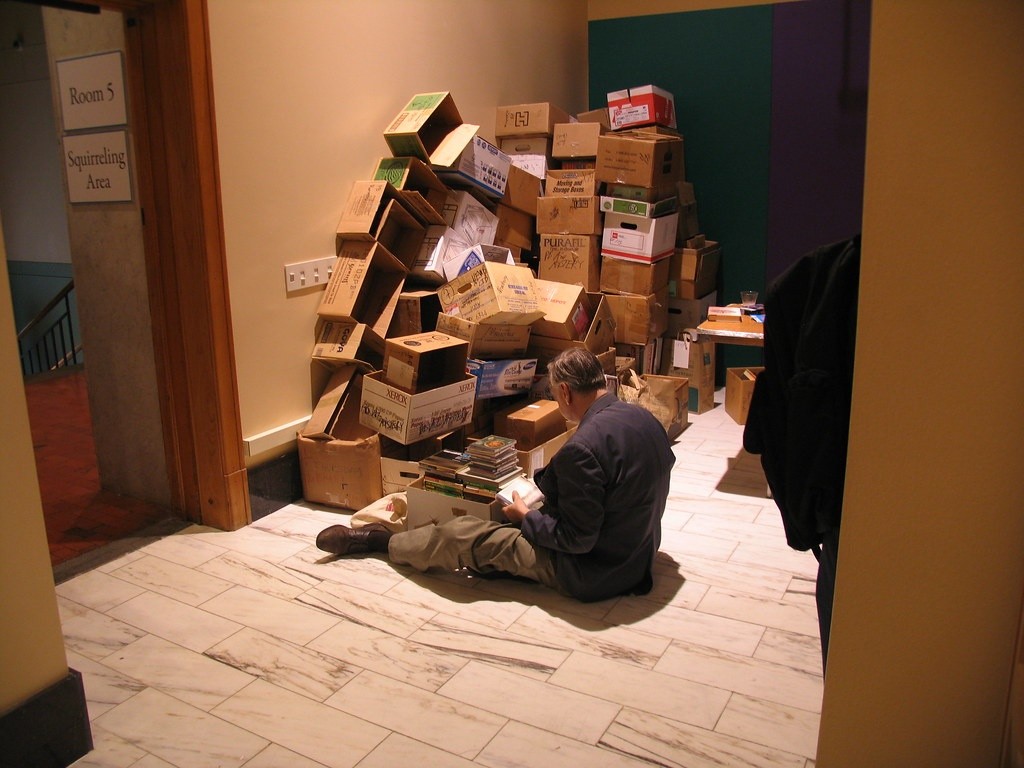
[316,522,390,554]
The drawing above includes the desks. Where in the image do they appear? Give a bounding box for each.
[697,303,764,346]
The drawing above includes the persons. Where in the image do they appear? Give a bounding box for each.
[316,347,676,602]
[742,235,862,682]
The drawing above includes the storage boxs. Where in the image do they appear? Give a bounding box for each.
[726,366,766,425]
[296,86,716,531]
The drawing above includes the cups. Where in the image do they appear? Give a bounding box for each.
[740,290,758,309]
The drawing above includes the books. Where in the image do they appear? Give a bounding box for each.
[495,476,545,509]
[418,434,528,504]
[707,306,742,322]
[750,315,765,323]
[740,303,765,314]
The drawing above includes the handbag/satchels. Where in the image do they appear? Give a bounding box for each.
[351,489,408,532]
[743,366,845,553]
